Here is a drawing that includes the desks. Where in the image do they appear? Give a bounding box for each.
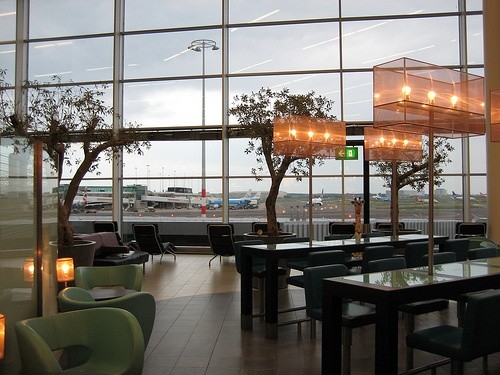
[322,257,500,375]
[93,285,125,299]
[240,233,449,332]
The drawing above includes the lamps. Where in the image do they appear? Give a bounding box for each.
[372,57,486,139]
[24,259,35,288]
[56,257,74,288]
[363,127,423,163]
[0,315,5,360]
[273,115,346,159]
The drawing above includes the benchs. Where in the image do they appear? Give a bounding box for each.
[72,232,149,276]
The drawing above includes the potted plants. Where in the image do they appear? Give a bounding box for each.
[225,87,337,289]
[0,67,152,268]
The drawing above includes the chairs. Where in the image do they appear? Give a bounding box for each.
[207,220,500,375]
[14,221,178,375]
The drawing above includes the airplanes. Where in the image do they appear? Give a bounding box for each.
[206,188,262,208]
[371,191,384,200]
[417,195,440,204]
[303,189,324,207]
[449,189,474,201]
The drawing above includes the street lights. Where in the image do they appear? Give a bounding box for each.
[187,39,218,217]
[135,165,176,192]
